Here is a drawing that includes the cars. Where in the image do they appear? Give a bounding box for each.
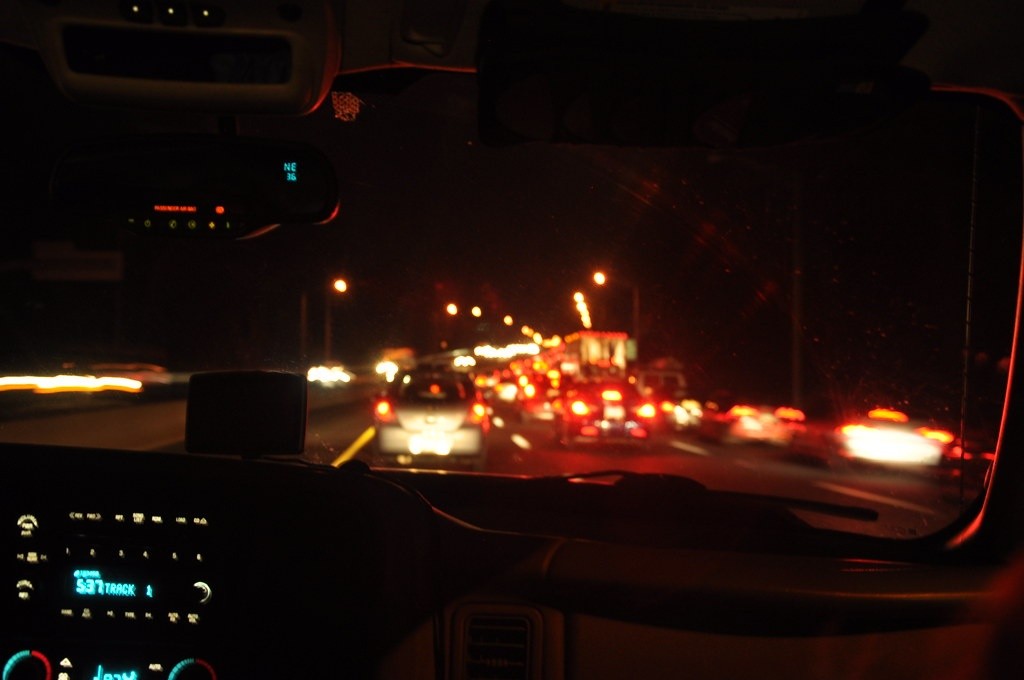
[302,327,957,489]
